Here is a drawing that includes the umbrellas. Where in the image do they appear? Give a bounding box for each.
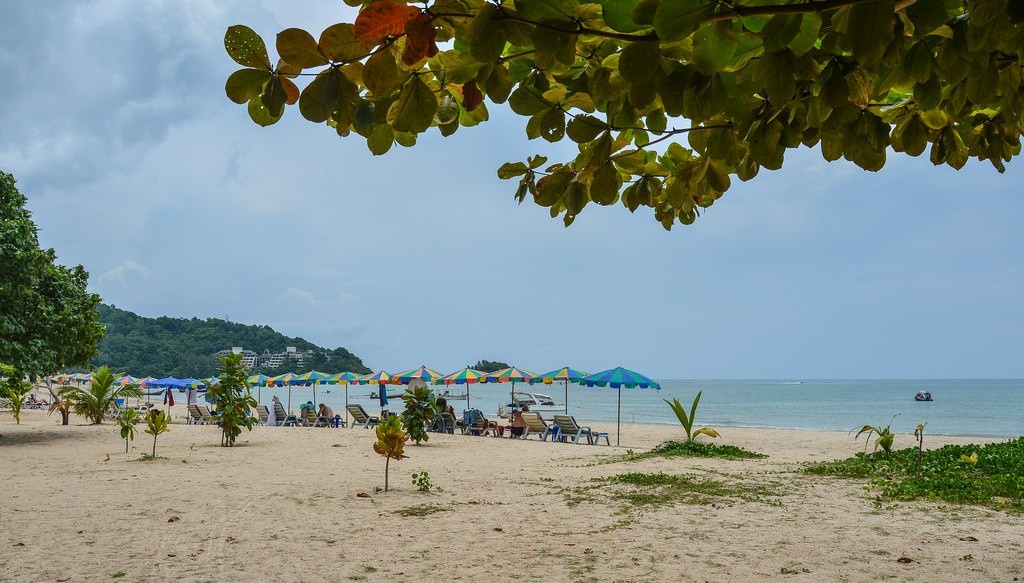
[579,365,662,446]
[431,366,490,410]
[290,370,333,410]
[265,371,300,415]
[247,373,270,404]
[480,366,537,437]
[314,369,364,428]
[33,372,219,418]
[350,369,395,410]
[389,365,446,383]
[529,366,593,416]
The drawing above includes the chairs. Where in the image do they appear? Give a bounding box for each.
[431,413,464,434]
[187,405,209,425]
[197,405,219,425]
[256,405,271,426]
[520,412,575,442]
[299,404,343,428]
[23,402,51,410]
[346,404,381,429]
[552,415,610,445]
[273,403,301,427]
[106,405,149,422]
[463,409,511,437]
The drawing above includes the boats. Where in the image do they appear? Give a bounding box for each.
[369,384,406,400]
[441,390,467,400]
[913,390,934,401]
[140,388,166,396]
[498,389,566,413]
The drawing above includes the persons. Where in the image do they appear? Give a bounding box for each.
[151,408,161,420]
[445,406,456,421]
[510,404,530,439]
[306,401,311,404]
[30,394,49,405]
[317,403,335,427]
